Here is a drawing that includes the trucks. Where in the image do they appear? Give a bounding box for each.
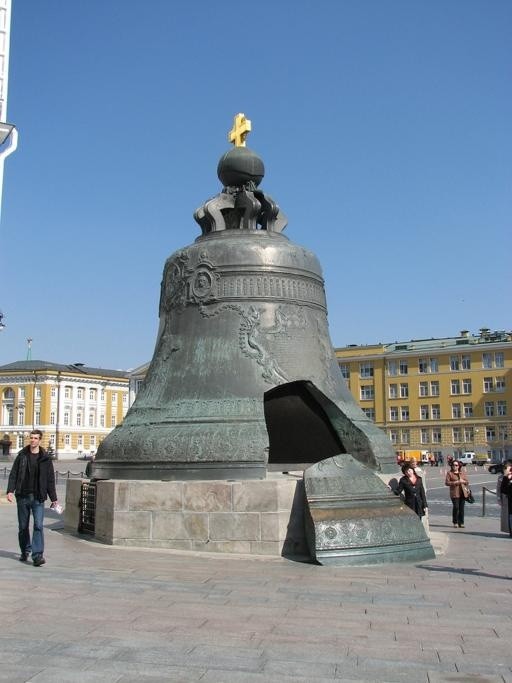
[396,449,431,466]
[456,452,491,466]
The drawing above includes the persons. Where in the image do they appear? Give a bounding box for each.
[496,461,512,537]
[386,463,428,521]
[7,430,58,566]
[444,459,470,528]
[395,452,453,467]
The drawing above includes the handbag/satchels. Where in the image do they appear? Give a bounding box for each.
[465,491,475,503]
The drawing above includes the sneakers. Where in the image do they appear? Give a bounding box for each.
[454,524,465,528]
[19,552,29,560]
[33,556,46,566]
